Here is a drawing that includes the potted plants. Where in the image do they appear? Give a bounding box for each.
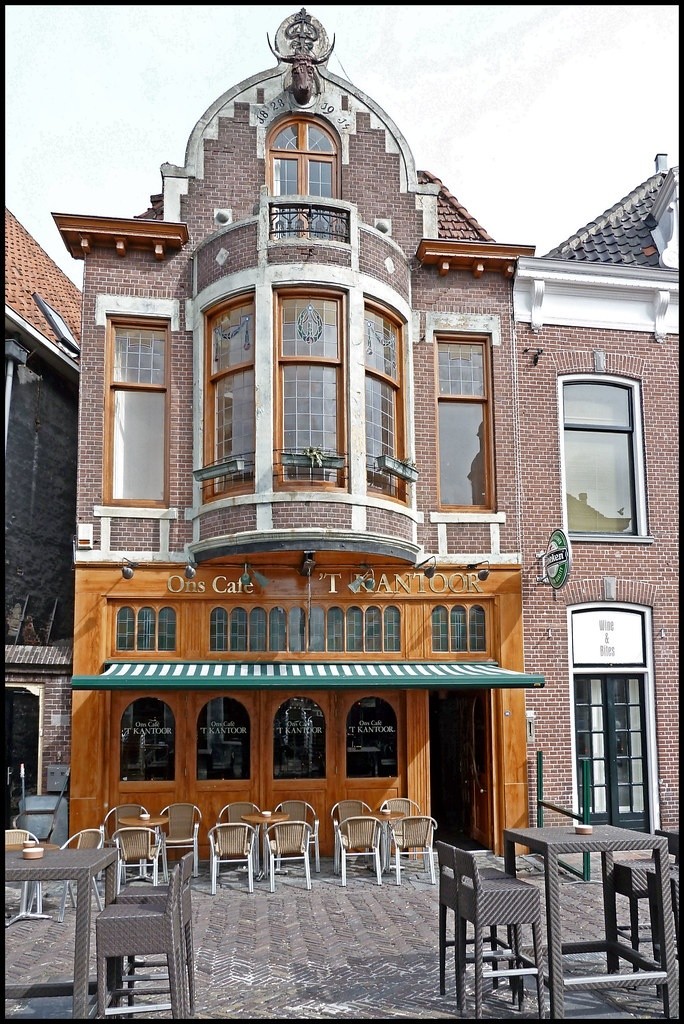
[280,446,345,469]
[376,454,419,482]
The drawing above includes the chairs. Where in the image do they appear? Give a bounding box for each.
[435,840,517,1006]
[95,863,183,1019]
[5,798,438,927]
[647,865,680,998]
[116,850,196,1018]
[613,830,679,990]
[455,848,545,1020]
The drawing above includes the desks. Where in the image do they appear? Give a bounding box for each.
[241,811,289,880]
[363,811,406,874]
[5,847,119,1018]
[118,815,171,884]
[503,824,679,1019]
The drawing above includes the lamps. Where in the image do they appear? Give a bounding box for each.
[122,557,491,581]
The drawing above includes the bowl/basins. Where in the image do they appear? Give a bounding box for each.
[382,810,390,814]
[262,811,271,817]
[23,848,43,859]
[140,814,150,820]
[23,841,35,848]
[575,825,592,834]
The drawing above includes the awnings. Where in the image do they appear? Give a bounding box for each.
[72,659,545,690]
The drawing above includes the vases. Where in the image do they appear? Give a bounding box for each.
[192,459,245,482]
